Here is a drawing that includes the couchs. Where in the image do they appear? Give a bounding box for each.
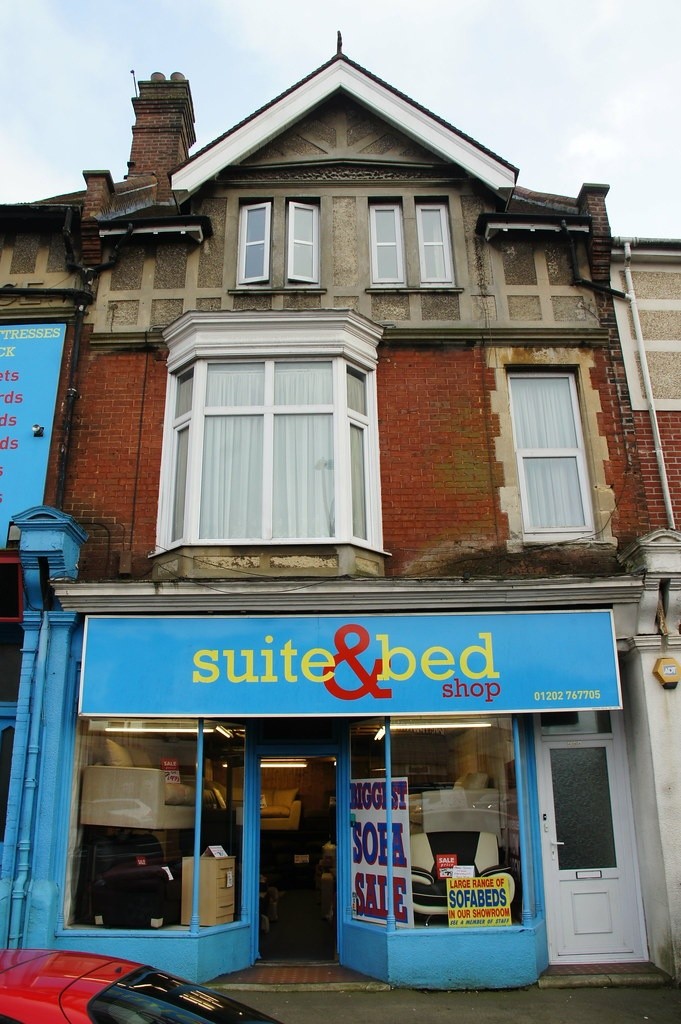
[408,772,502,839]
[80,764,302,831]
[77,834,181,928]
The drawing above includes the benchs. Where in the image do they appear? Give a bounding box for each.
[409,831,515,925]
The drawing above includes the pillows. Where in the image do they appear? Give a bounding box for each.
[104,738,134,766]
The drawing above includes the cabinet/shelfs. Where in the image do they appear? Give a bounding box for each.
[182,856,235,926]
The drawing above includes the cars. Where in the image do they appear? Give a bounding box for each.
[0,947,282,1024]
[76,835,187,930]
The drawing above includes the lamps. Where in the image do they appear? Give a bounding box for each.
[381,719,492,728]
[32,424,45,437]
[374,726,385,740]
[260,762,308,768]
[216,725,233,738]
